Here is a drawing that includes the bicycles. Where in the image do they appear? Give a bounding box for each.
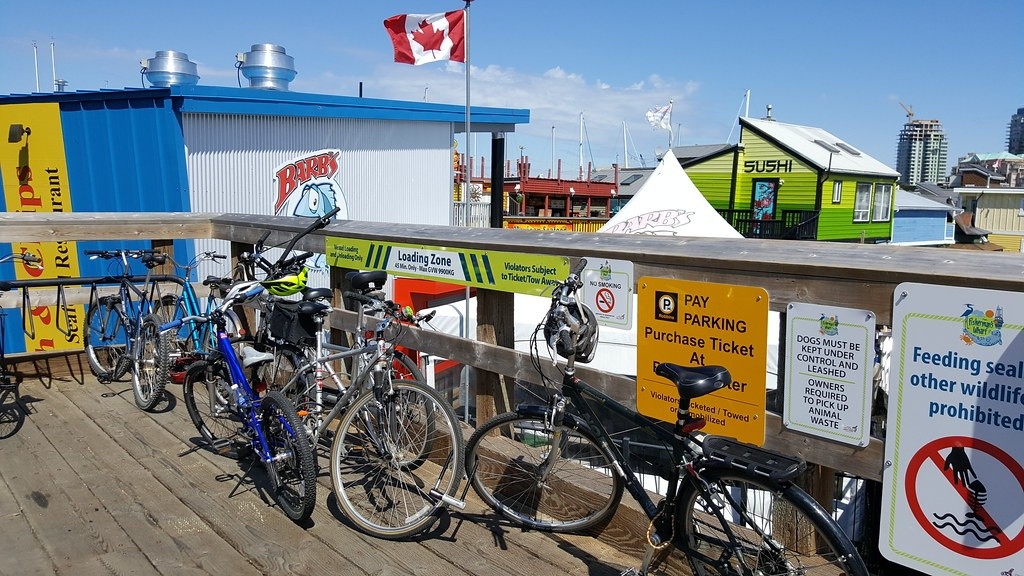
[82,208,467,541]
[466,259,868,576]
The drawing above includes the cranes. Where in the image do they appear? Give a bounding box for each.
[899,100,913,121]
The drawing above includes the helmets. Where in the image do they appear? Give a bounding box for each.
[259,266,308,296]
[543,301,599,363]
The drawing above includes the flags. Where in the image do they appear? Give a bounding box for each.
[646,104,673,144]
[384,8,467,67]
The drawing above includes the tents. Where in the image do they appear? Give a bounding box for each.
[595,150,746,238]
